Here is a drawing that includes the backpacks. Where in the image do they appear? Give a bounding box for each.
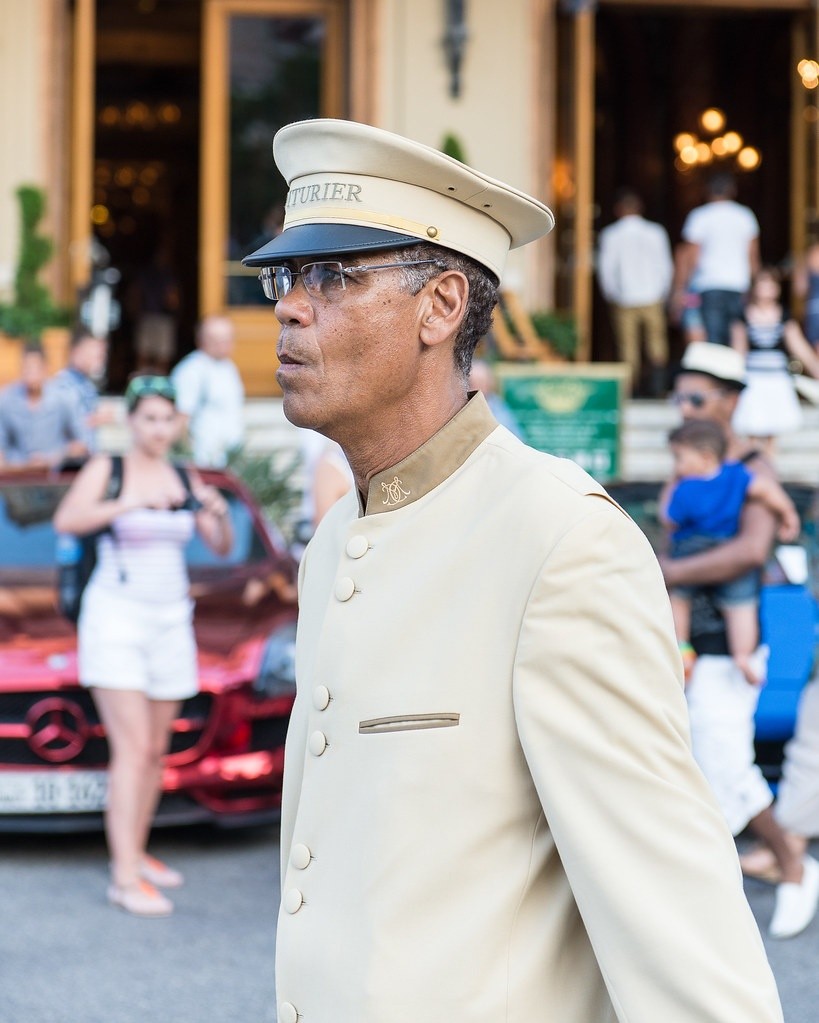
[56,453,196,621]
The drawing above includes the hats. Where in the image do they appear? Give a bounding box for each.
[241,119,554,290]
[682,343,747,391]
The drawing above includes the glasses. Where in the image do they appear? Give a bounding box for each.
[124,378,176,411]
[258,258,450,301]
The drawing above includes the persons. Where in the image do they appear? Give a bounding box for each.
[241,119,818,1022]
[169,316,247,474]
[307,164,818,938]
[0,328,109,479]
[53,374,232,919]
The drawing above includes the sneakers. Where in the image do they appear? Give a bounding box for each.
[769,858,818,937]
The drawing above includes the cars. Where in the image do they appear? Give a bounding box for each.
[1,462,307,836]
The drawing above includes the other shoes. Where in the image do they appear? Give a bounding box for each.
[108,881,171,916]
[739,850,773,884]
[135,853,182,888]
